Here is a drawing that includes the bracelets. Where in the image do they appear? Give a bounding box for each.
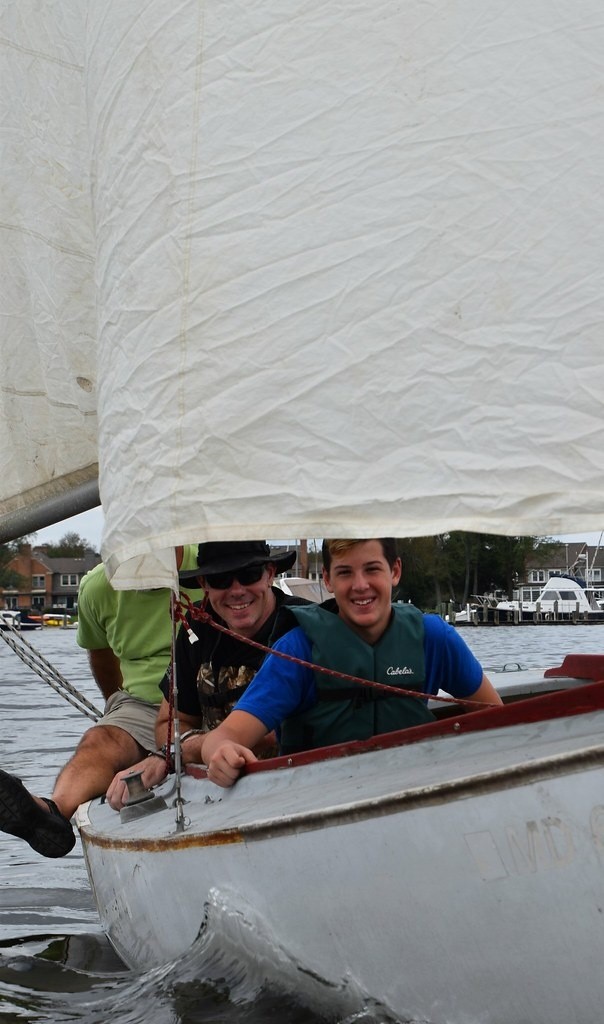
[180,728,207,742]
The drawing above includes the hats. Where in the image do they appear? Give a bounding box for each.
[178,540,297,589]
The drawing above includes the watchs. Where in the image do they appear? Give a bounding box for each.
[161,742,183,766]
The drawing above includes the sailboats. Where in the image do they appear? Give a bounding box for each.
[1,1,604,1024]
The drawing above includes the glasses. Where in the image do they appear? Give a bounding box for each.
[206,562,263,589]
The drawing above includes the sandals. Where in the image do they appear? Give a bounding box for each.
[0,769,76,858]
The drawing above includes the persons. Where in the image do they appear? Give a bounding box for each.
[200,534,504,789]
[0,542,204,857]
[105,539,314,812]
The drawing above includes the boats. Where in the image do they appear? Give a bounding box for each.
[476,576,604,623]
